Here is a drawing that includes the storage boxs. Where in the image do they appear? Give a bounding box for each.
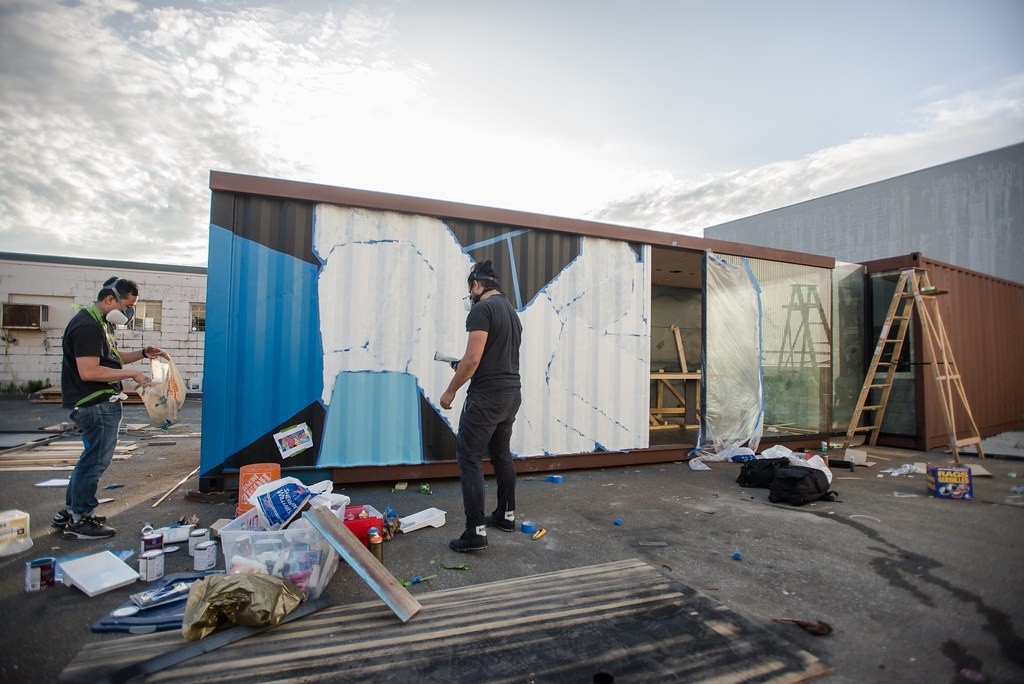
[927,463,975,500]
[0,510,33,556]
[216,488,352,602]
[342,504,384,550]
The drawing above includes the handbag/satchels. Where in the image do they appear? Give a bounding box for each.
[137,350,187,430]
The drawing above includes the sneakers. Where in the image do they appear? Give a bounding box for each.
[450,524,490,552]
[60,515,117,538]
[483,508,516,535]
[53,510,107,529]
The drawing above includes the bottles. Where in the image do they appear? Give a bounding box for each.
[368,526,379,550]
[821,442,828,468]
[369,533,383,565]
[850,457,855,472]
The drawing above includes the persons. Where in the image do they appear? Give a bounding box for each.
[49,275,171,541]
[438,260,524,553]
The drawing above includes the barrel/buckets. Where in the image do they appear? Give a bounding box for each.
[140,533,163,554]
[0,509,33,557]
[194,540,217,571]
[235,463,280,519]
[139,549,164,582]
[189,529,210,556]
[24,557,56,592]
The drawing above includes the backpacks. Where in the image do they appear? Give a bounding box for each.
[769,468,835,505]
[736,458,789,488]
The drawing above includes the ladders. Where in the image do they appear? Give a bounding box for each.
[771,284,849,428]
[843,266,984,463]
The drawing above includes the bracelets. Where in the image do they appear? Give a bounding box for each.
[142,349,148,359]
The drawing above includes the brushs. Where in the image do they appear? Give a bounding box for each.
[434,350,461,368]
